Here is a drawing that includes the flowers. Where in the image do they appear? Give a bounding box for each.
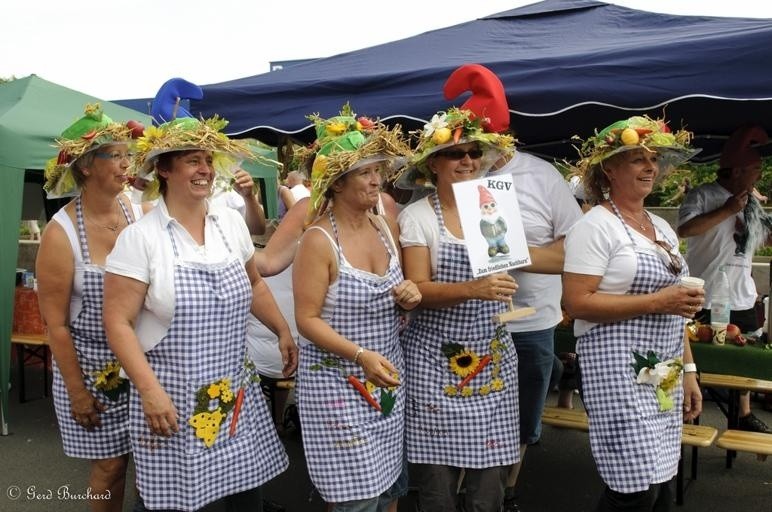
[185,374,237,449]
[629,348,684,413]
[423,112,447,139]
[91,357,131,403]
[324,120,347,139]
[440,320,513,401]
[135,124,164,154]
[365,363,399,418]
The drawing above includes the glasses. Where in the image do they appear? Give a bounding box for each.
[433,149,484,161]
[87,149,135,161]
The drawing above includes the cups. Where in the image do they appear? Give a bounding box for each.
[711,322,727,344]
[755,295,769,333]
[680,275,707,316]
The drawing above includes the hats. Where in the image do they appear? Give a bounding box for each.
[394,111,512,190]
[303,130,409,226]
[147,77,204,128]
[46,112,141,201]
[127,116,248,194]
[444,64,510,135]
[720,125,769,168]
[583,114,704,202]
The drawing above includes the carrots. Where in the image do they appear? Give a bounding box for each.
[348,374,381,410]
[229,388,243,435]
[454,128,461,145]
[611,129,652,136]
[457,354,492,389]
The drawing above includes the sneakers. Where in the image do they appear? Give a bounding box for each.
[223,493,286,511]
[503,487,522,512]
[738,413,771,433]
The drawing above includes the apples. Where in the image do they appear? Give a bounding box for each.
[126,120,144,139]
[696,326,713,342]
[725,324,741,340]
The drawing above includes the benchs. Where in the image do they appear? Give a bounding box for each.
[714,427,772,458]
[697,369,771,471]
[454,399,719,505]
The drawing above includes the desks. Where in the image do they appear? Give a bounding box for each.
[689,335,772,471]
[10,331,53,405]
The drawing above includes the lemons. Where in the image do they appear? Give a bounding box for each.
[431,128,451,145]
[621,129,639,145]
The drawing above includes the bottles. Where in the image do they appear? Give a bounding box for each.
[710,266,731,324]
[16,220,40,290]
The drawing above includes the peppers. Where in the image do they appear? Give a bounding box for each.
[81,130,96,139]
